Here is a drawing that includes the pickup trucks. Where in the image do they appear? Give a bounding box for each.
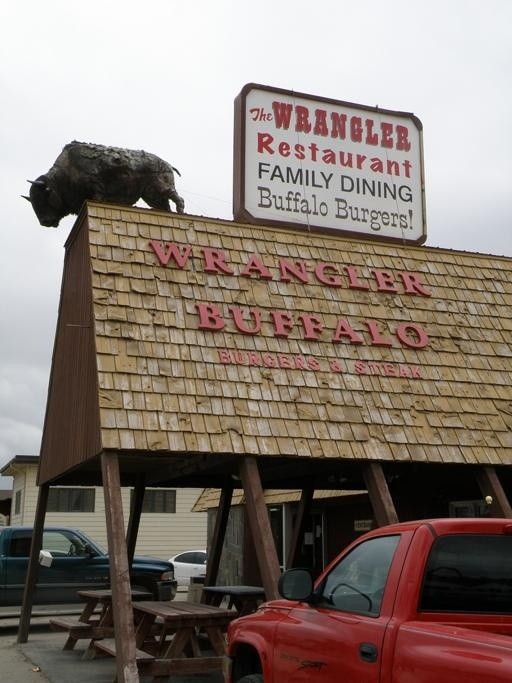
[218,514,511,682]
[0,524,178,608]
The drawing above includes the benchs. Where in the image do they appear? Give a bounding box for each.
[49,583,265,682]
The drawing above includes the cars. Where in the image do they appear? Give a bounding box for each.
[170,550,209,587]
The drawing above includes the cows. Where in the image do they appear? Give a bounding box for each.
[19,139,185,228]
